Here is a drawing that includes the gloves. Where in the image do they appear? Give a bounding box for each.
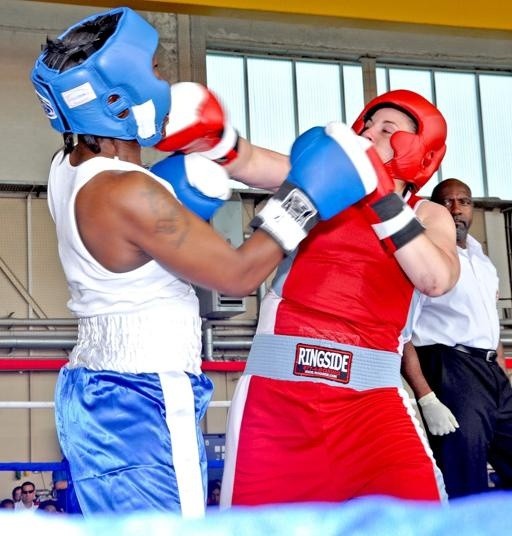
[250,123,427,259]
[418,391,461,437]
[148,154,233,223]
[155,79,243,168]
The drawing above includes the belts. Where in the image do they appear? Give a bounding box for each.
[452,343,499,362]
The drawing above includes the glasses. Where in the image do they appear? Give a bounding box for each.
[20,489,34,494]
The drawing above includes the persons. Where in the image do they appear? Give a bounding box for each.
[402,179,511,499]
[151,80,461,508]
[29,7,378,512]
[0,478,221,513]
